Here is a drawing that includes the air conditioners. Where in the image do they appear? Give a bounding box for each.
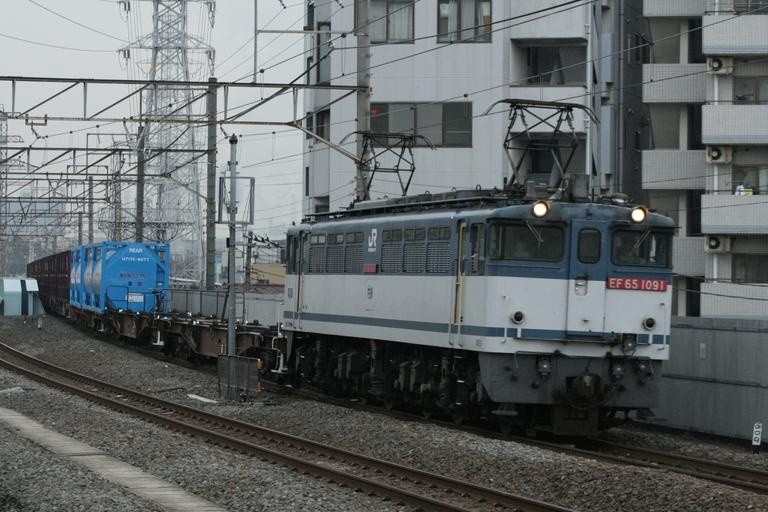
[707,55,733,77]
[706,145,732,164]
[704,234,730,256]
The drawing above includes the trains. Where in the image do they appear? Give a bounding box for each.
[27,187,681,437]
